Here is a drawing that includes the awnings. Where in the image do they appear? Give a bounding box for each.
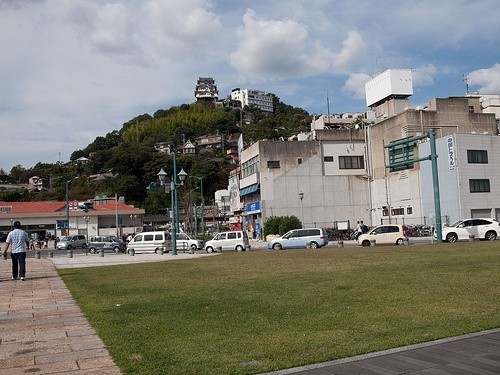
[239,184,258,195]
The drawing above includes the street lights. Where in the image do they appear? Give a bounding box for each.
[66,175,80,237]
[189,174,206,236]
[299,191,304,228]
[157,155,188,254]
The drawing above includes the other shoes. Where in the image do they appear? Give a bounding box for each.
[11,277,16,279]
[21,277,25,281]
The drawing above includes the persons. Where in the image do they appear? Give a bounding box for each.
[3,221,30,281]
[30,232,60,250]
[123,233,133,242]
[353,220,366,242]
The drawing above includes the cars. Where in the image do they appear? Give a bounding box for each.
[433,217,500,243]
[356,224,409,246]
[266,227,329,250]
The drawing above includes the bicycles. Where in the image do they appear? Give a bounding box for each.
[324,225,432,240]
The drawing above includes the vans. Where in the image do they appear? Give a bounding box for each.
[88,235,126,252]
[205,230,252,252]
[168,233,204,250]
[126,230,173,253]
[56,234,86,250]
